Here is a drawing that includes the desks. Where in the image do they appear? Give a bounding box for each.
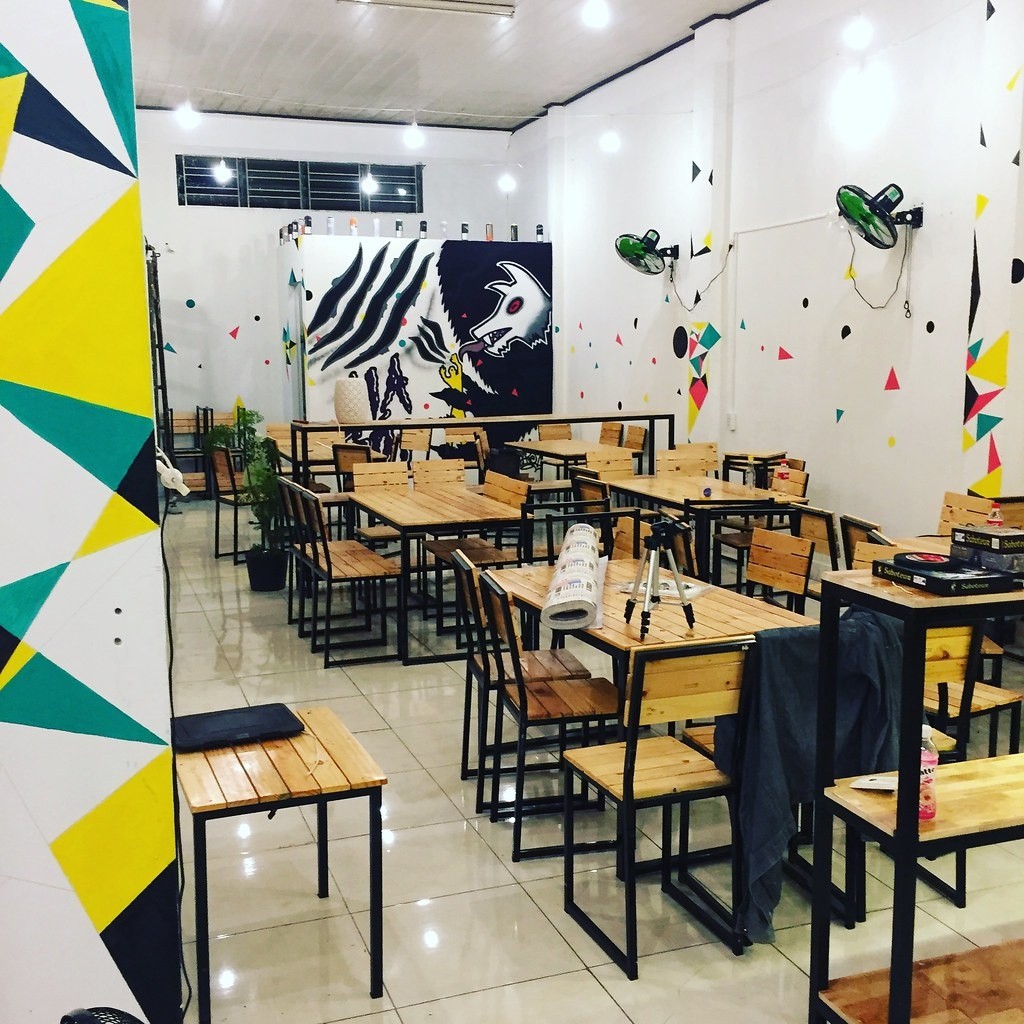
[603,475,810,587]
[481,558,820,749]
[503,439,643,514]
[347,482,534,666]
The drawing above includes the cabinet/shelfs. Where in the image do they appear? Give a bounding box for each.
[808,570,1024,1024]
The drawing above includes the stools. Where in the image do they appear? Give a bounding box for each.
[167,706,389,1024]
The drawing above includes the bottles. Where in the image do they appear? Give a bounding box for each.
[987,503,1004,526]
[919,723,939,822]
[778,458,789,496]
[746,455,755,496]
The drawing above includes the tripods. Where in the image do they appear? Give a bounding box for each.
[624,521,695,640]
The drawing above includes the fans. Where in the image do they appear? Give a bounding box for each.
[615,230,679,276]
[836,183,923,250]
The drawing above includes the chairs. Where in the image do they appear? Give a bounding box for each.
[166,412,1024,983]
